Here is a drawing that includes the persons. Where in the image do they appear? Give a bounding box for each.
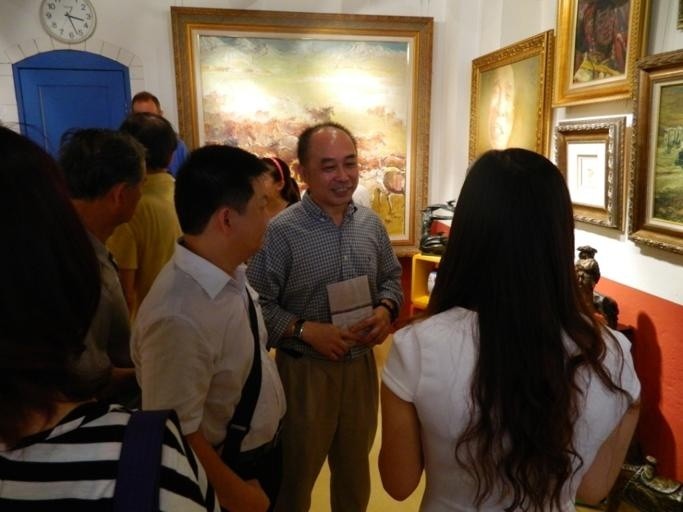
[574,1,627,84]
[379,150,641,512]
[483,65,518,149]
[245,122,405,512]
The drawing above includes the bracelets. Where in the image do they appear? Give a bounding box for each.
[373,301,396,325]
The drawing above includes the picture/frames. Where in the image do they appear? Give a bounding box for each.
[170,6,436,261]
[553,0,651,107]
[553,116,626,233]
[625,47,683,256]
[467,30,554,187]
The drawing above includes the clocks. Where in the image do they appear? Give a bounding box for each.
[37,0,96,42]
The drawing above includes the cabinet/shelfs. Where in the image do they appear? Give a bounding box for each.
[409,248,440,326]
